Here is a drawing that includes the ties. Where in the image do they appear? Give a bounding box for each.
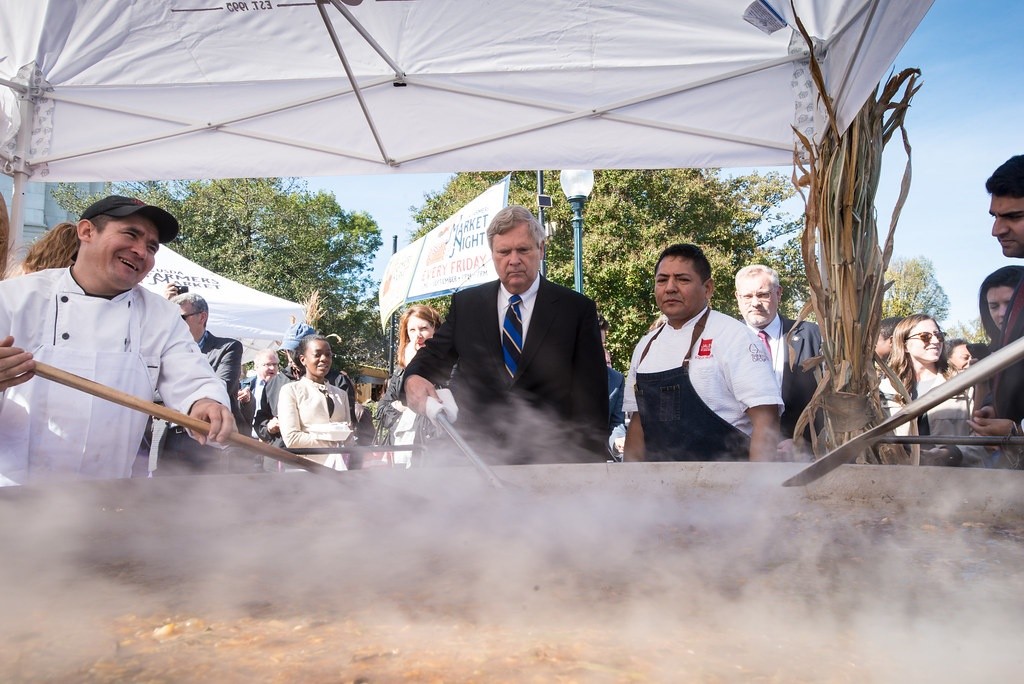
[758,330,772,355]
[503,295,522,384]
[260,381,268,411]
[992,281,1024,418]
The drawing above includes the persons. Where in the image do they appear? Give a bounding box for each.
[599,312,625,441]
[377,305,448,464]
[620,244,786,464]
[734,265,821,456]
[22,223,79,273]
[401,207,609,467]
[238,322,375,474]
[139,280,243,474]
[0,195,237,487]
[874,156,1024,470]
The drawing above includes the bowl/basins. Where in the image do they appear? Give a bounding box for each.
[308,424,354,441]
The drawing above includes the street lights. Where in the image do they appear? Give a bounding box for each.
[559,169,594,294]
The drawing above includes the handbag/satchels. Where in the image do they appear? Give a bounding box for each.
[356,406,392,471]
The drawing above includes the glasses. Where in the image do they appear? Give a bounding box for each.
[181,312,201,320]
[258,362,278,367]
[905,332,947,342]
[737,288,775,300]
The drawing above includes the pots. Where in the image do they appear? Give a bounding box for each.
[0,459,1022,683]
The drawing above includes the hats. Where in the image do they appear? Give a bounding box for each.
[81,195,179,243]
[279,322,315,350]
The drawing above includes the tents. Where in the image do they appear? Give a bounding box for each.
[139,242,306,364]
[0,0,932,274]
